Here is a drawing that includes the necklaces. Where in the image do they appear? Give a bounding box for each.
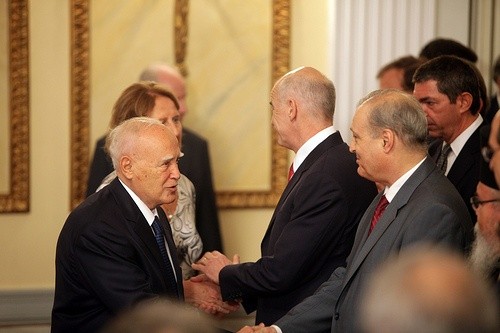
[164,205,174,220]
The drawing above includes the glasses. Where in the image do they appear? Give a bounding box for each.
[470,197,500,211]
[482,148,496,162]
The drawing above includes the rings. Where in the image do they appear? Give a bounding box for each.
[202,258,207,263]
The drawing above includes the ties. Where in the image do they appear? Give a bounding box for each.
[436,144,452,175]
[153,216,177,290]
[368,195,389,236]
[288,164,294,181]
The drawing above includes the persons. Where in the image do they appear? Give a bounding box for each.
[377,56,419,95]
[357,243,500,333]
[253,89,471,333]
[192,65,379,327]
[51,118,264,333]
[86,66,222,259]
[97,81,203,279]
[110,299,213,333]
[419,38,478,63]
[474,54,500,291]
[413,55,492,222]
